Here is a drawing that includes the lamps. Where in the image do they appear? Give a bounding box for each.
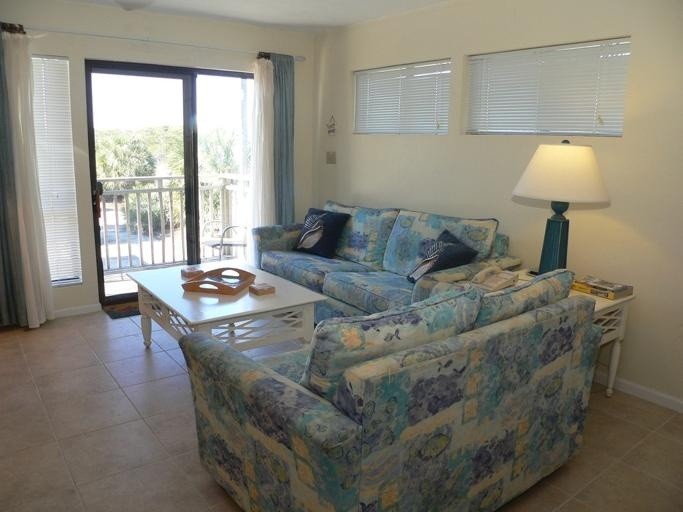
[512,140,608,275]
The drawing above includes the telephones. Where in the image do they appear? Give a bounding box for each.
[472,265,518,290]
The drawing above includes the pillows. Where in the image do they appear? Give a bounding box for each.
[292,208,351,259]
[407,229,480,285]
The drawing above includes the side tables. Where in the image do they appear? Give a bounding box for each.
[512,269,634,397]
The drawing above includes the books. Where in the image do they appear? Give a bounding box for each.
[571,274,633,300]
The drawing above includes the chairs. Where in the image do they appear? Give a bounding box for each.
[201,220,247,260]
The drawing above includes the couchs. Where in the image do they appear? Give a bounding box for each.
[177,269,605,512]
[250,200,523,326]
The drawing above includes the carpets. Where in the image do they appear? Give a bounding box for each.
[102,299,160,319]
